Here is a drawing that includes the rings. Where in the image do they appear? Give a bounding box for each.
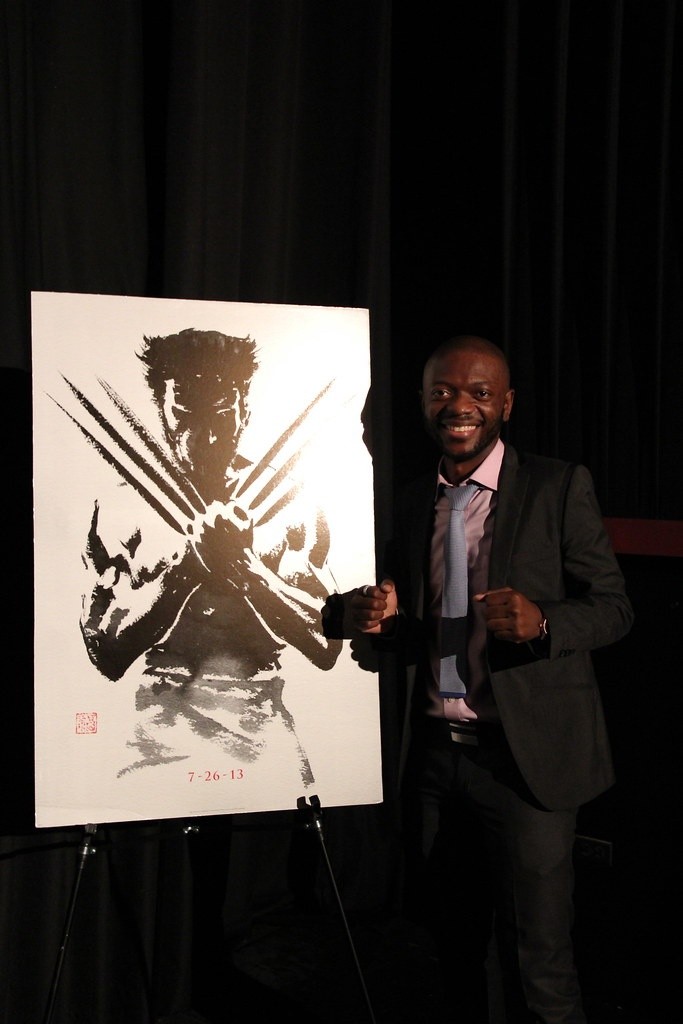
[363,585,369,596]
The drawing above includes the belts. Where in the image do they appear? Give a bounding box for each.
[416,714,504,751]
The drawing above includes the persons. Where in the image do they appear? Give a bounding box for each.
[350,335,637,1024]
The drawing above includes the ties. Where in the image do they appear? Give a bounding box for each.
[438,485,479,697]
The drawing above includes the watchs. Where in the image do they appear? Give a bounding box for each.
[536,617,550,642]
